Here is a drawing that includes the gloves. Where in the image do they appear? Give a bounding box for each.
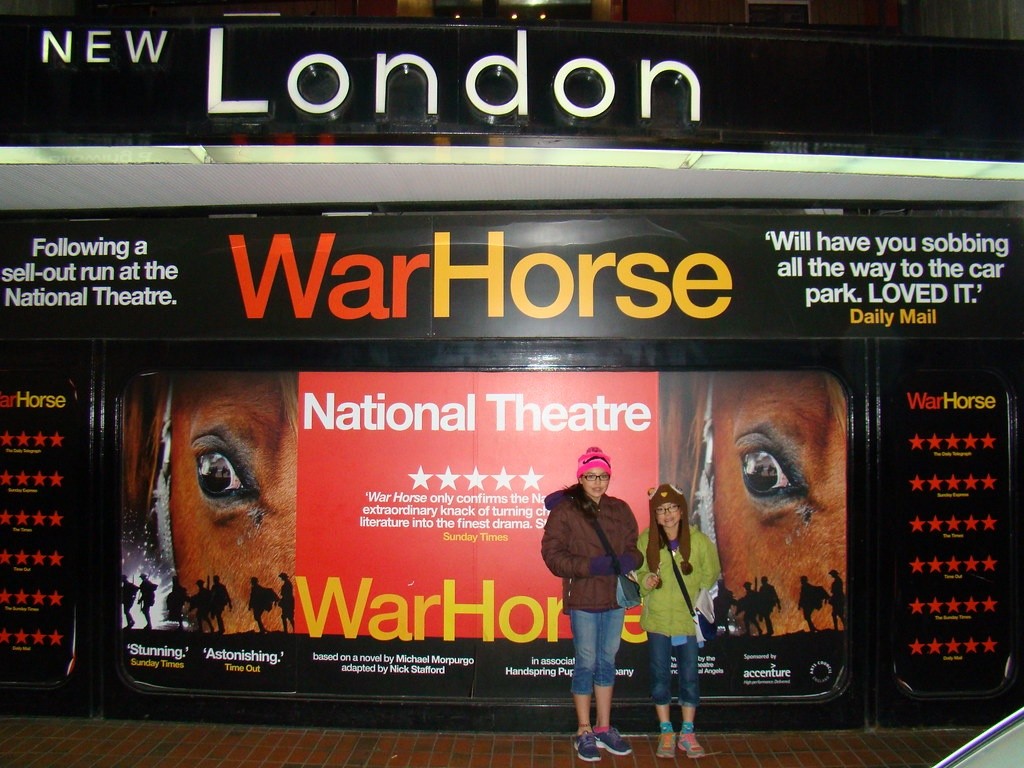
[589,556,616,576]
[619,552,635,574]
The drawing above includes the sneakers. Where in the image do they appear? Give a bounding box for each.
[591,725,632,757]
[574,730,602,761]
[678,731,705,758]
[656,732,676,758]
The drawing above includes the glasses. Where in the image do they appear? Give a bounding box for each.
[655,503,679,515]
[581,475,610,481]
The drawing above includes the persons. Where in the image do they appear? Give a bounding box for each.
[541,447,644,762]
[637,483,720,758]
[711,569,844,637]
[120,568,296,633]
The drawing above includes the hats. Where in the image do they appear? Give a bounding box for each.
[828,570,839,574]
[139,573,147,577]
[122,575,128,579]
[576,447,612,478]
[278,573,289,578]
[195,579,205,583]
[647,484,693,589]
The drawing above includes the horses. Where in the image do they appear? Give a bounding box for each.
[658,371,847,637]
[122,371,295,634]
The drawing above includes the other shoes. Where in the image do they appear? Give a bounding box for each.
[124,621,135,629]
[144,624,152,630]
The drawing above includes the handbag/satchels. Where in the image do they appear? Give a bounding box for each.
[692,587,717,642]
[616,574,641,609]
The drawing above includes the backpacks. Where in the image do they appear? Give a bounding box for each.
[806,583,823,610]
[258,585,277,612]
